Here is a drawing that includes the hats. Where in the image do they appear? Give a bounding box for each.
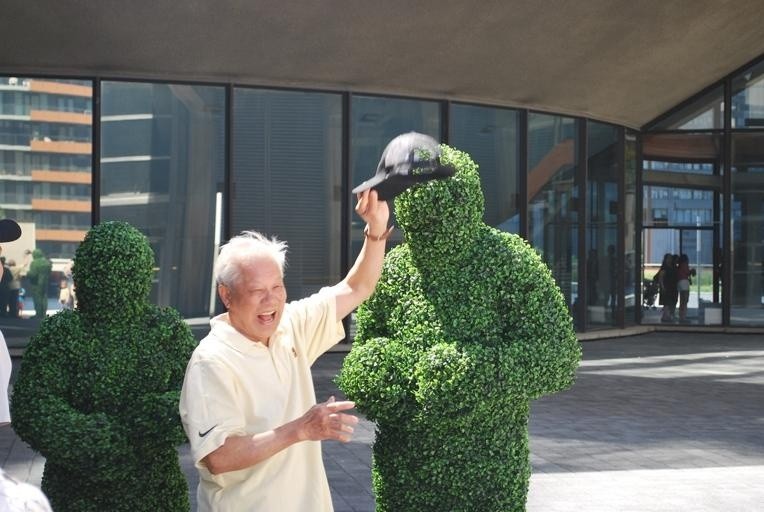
[351,129,458,202]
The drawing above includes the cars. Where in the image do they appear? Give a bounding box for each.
[17,258,75,311]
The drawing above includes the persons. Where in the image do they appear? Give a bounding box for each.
[58,279,73,310]
[176,178,392,509]
[0,257,13,318]
[575,243,633,315]
[4,248,31,319]
[676,254,693,323]
[671,254,679,317]
[656,253,676,322]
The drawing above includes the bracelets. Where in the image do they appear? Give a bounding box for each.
[363,222,389,242]
